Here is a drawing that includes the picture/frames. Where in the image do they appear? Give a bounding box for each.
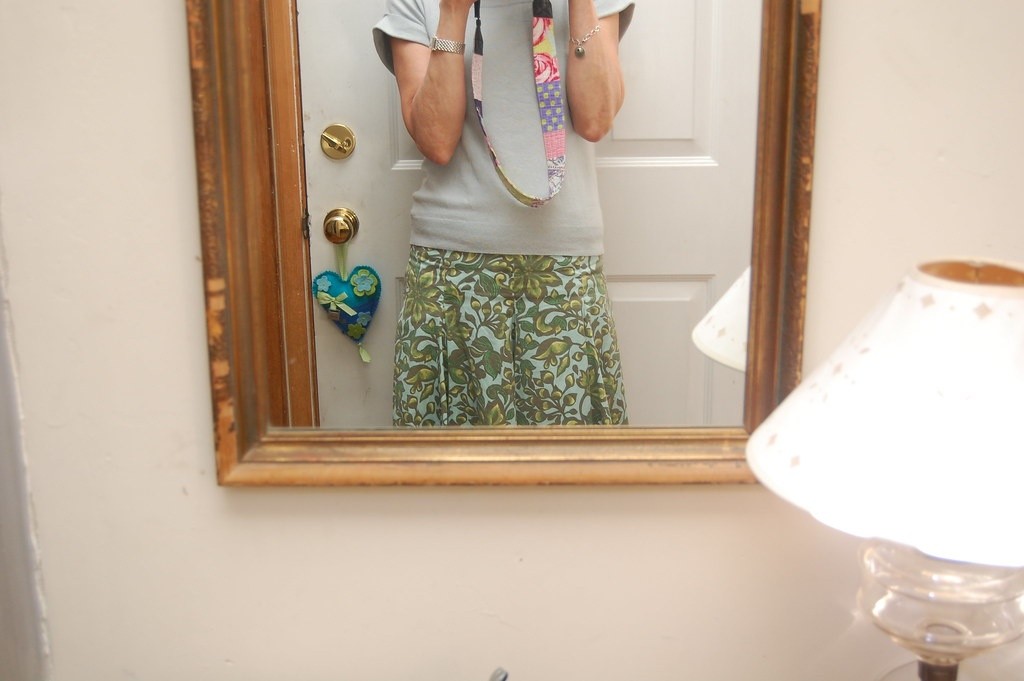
[185,0,824,487]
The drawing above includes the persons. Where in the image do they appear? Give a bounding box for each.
[373,1,636,427]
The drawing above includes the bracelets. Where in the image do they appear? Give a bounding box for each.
[431,36,465,55]
[570,25,600,56]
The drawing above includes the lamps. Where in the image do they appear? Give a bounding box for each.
[743,260,1024,681]
